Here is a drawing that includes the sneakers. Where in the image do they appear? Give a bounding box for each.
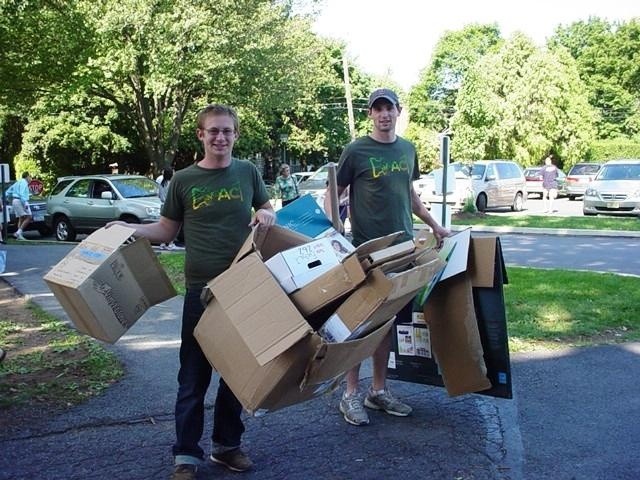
[339,388,370,426]
[171,464,198,480]
[15,230,26,240]
[210,447,253,472]
[363,385,413,417]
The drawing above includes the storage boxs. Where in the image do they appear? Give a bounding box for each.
[45,225,178,346]
[194,189,513,418]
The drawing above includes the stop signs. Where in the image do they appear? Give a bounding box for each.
[28,180,44,196]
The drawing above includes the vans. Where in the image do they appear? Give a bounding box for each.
[420,159,529,213]
[566,160,605,204]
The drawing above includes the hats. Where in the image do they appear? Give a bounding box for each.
[368,89,399,109]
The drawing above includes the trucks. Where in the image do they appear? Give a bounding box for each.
[0,180,53,239]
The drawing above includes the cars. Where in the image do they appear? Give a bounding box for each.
[291,156,567,208]
[582,158,640,217]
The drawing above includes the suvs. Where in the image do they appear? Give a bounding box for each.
[44,172,167,243]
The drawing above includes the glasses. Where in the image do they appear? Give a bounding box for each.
[199,128,236,136]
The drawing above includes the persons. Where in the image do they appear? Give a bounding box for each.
[331,239,351,262]
[105,102,276,479]
[122,162,183,251]
[5,172,32,242]
[533,157,559,213]
[325,179,349,236]
[322,89,452,425]
[272,164,301,207]
